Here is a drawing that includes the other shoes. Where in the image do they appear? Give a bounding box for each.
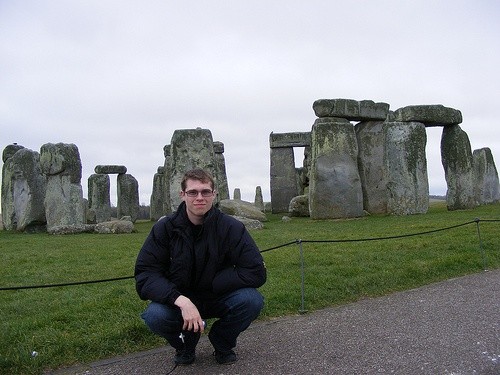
[213,348,236,364]
[173,336,200,365]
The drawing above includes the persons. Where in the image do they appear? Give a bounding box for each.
[134,168,267,364]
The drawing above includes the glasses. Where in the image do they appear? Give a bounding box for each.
[184,190,213,196]
